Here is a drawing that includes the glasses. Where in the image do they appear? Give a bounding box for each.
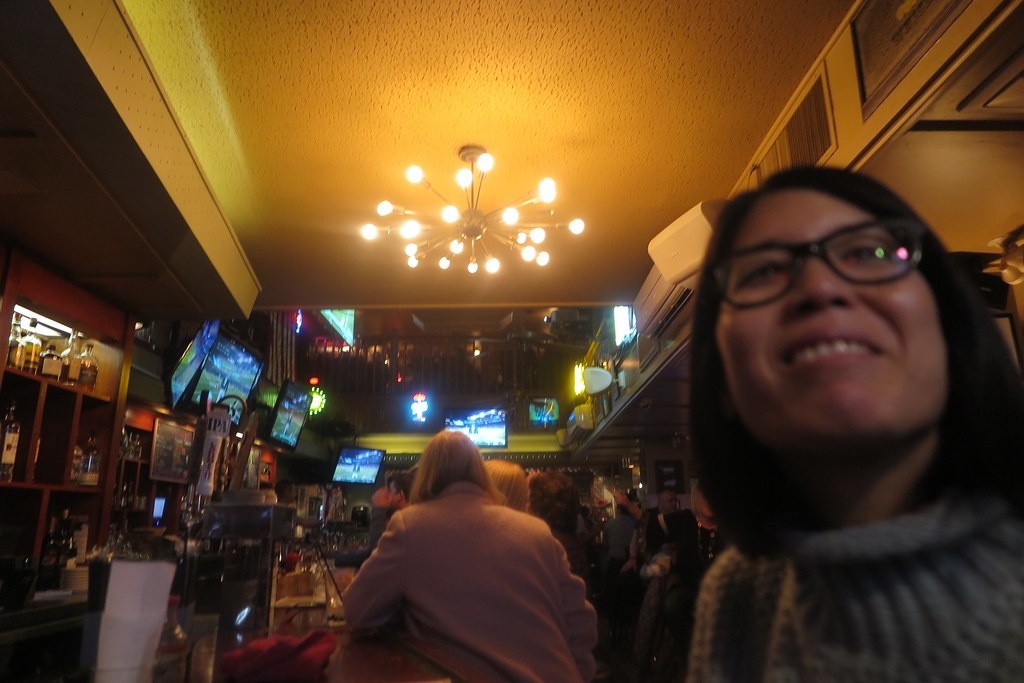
[713,221,922,309]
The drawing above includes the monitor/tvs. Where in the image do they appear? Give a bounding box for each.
[441,405,508,449]
[529,397,559,429]
[262,378,313,451]
[328,445,386,487]
[183,326,266,429]
[164,316,220,409]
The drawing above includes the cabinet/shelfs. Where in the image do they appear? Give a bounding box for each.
[1,244,348,610]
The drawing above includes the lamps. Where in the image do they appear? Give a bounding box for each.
[360,146,585,274]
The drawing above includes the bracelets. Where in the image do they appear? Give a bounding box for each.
[629,556,637,562]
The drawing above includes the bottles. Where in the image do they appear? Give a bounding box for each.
[59,327,82,386]
[77,429,101,486]
[15,318,42,375]
[35,343,62,380]
[7,312,22,367]
[0,398,22,483]
[39,508,74,591]
[77,343,99,391]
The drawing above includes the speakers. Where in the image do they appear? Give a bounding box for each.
[647,197,728,285]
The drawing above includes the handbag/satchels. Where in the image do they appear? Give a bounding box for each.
[219,628,337,680]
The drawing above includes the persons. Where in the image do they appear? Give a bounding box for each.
[618,465,707,683]
[602,505,638,646]
[204,441,215,479]
[342,429,599,683]
[369,487,389,550]
[352,461,362,478]
[369,471,413,555]
[687,162,1023,683]
[282,409,294,436]
[263,463,270,473]
[215,370,230,403]
[482,458,528,514]
[229,399,238,418]
[580,504,594,533]
[530,469,591,595]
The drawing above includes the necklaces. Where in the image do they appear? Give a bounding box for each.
[696,522,719,564]
